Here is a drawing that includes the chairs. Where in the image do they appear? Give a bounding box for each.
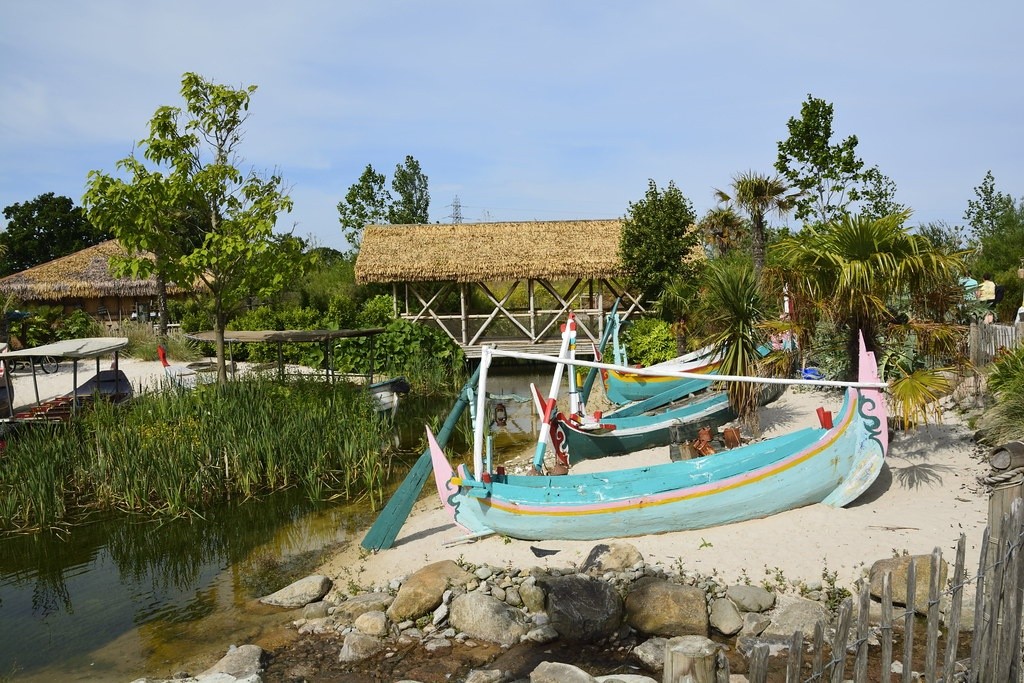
[16,393,128,424]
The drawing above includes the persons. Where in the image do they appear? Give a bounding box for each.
[957,271,979,305]
[978,274,995,307]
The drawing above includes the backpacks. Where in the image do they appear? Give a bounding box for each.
[993,280,1004,305]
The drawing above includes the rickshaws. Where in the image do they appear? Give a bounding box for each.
[0,312,58,374]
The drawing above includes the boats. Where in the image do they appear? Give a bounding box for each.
[183,327,409,412]
[424,329,893,540]
[0,337,132,458]
[530,329,800,468]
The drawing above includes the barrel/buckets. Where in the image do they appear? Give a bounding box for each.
[368,376,408,411]
[802,366,824,380]
